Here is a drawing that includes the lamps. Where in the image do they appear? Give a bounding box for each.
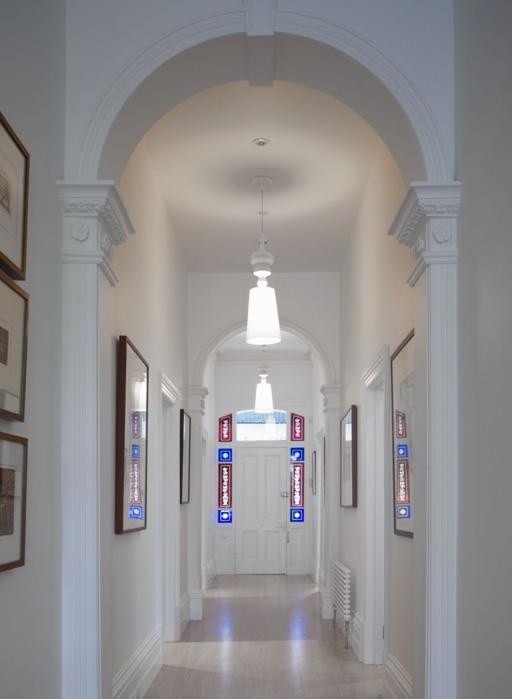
[246,176,281,346]
[254,369,273,414]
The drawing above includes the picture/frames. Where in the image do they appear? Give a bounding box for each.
[388,329,414,539]
[0,110,31,571]
[116,335,150,534]
[340,405,357,508]
[180,408,191,504]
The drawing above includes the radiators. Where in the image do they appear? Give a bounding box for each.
[331,560,353,648]
